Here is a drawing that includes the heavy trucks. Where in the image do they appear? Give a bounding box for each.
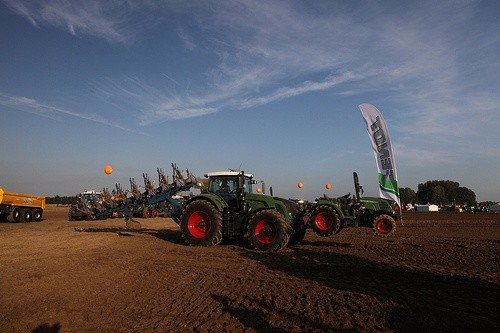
[0,189,47,224]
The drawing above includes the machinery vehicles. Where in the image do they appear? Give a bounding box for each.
[306,172,401,238]
[67,160,308,253]
[65,192,158,220]
